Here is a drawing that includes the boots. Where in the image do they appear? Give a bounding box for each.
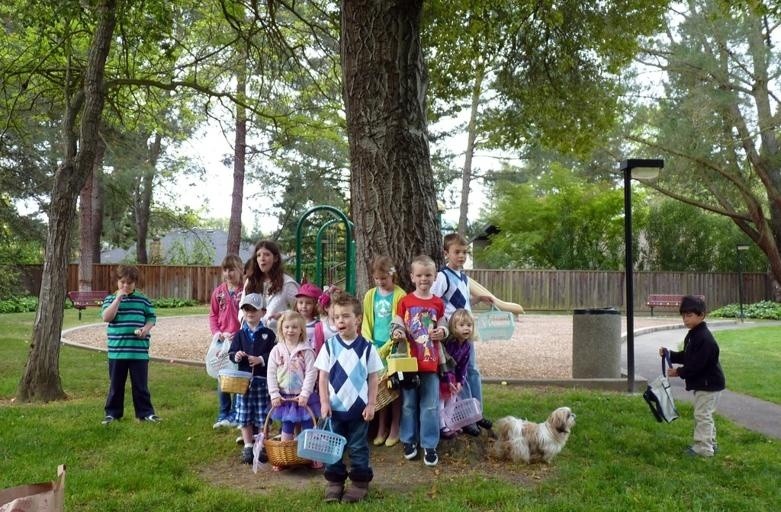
[342,467,374,503]
[323,464,348,502]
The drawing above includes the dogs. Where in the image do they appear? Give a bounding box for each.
[485,406,578,466]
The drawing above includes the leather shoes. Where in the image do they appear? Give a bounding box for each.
[374,430,400,447]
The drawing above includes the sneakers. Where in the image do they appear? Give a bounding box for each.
[403,443,418,460]
[211,416,244,432]
[144,414,162,424]
[235,434,258,444]
[235,442,269,465]
[440,417,493,440]
[424,448,439,466]
[101,415,114,425]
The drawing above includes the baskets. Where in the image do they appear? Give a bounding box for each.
[263,398,329,467]
[296,416,348,464]
[217,352,254,394]
[440,390,483,431]
[474,302,517,342]
[374,364,401,412]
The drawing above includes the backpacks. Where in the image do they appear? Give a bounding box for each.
[643,345,681,424]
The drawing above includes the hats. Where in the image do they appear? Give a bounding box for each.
[294,282,324,298]
[241,292,268,311]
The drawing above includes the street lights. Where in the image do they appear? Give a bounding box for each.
[736,243,750,324]
[622,158,664,396]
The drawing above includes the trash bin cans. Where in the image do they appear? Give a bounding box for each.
[573,308,622,378]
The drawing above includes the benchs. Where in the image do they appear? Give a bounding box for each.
[68,288,111,320]
[644,292,705,318]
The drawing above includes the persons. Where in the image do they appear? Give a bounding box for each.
[312,294,385,504]
[659,294,726,457]
[100,264,161,426]
[209,233,495,471]
